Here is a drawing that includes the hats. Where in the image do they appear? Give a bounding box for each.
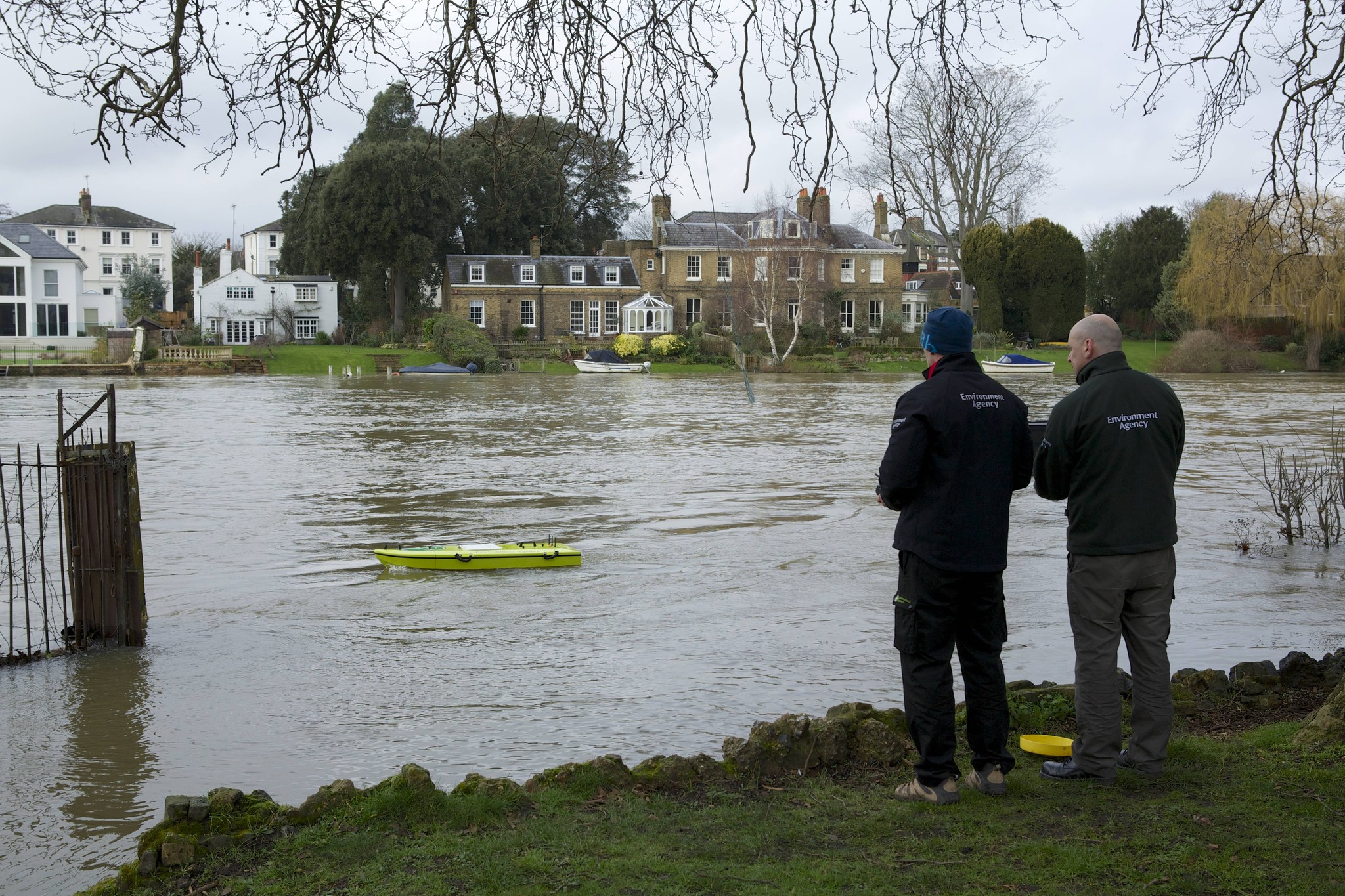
[920,307,974,356]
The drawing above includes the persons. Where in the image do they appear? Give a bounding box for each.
[1034,315,1187,780]
[875,307,1034,805]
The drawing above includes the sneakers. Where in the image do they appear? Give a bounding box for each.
[963,764,1007,796]
[894,774,960,805]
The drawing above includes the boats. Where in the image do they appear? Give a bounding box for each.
[373,538,584,572]
[982,354,1056,371]
[573,348,647,375]
[399,362,478,377]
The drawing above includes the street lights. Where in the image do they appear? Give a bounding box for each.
[191,288,202,342]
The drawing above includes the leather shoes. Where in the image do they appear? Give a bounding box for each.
[1117,748,1158,774]
[1039,756,1119,785]
